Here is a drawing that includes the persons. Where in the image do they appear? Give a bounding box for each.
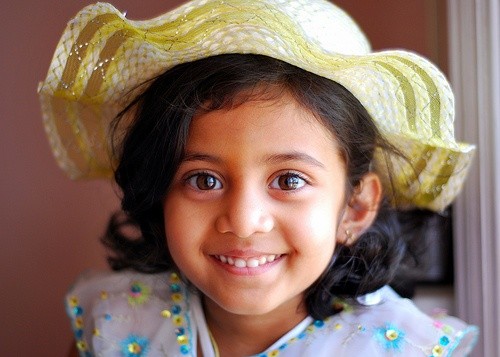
[34,1,483,357]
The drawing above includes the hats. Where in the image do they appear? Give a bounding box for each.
[37,1,476,215]
